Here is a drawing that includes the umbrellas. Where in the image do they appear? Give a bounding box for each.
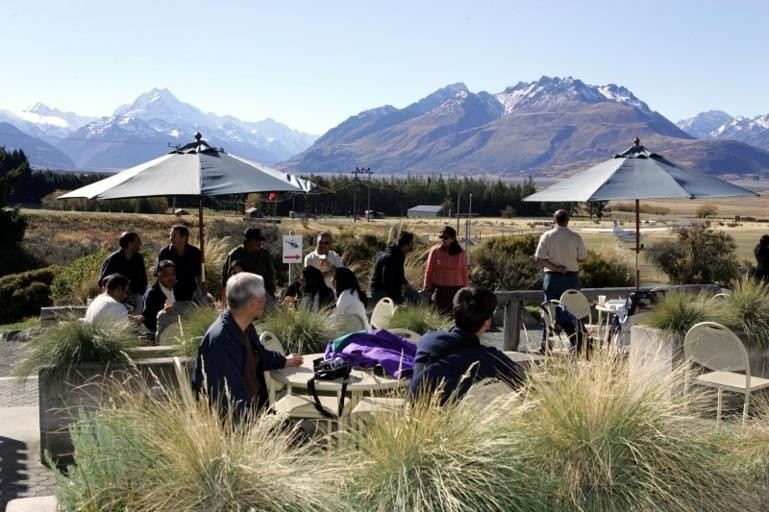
[59,131,333,297]
[519,136,761,298]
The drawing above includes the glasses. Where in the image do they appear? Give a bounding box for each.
[440,235,454,240]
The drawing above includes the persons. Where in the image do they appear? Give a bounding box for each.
[405,285,530,414]
[187,271,305,443]
[534,210,587,355]
[84,217,375,338]
[417,226,470,312]
[368,230,419,303]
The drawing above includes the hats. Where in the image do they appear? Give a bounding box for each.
[243,226,267,241]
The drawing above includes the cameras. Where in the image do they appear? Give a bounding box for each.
[313,357,351,380]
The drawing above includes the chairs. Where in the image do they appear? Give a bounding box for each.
[170,286,590,426]
[681,321,769,430]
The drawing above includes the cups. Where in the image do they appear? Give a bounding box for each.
[319,254,327,269]
[598,295,606,306]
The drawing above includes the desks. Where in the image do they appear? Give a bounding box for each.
[595,304,626,352]
